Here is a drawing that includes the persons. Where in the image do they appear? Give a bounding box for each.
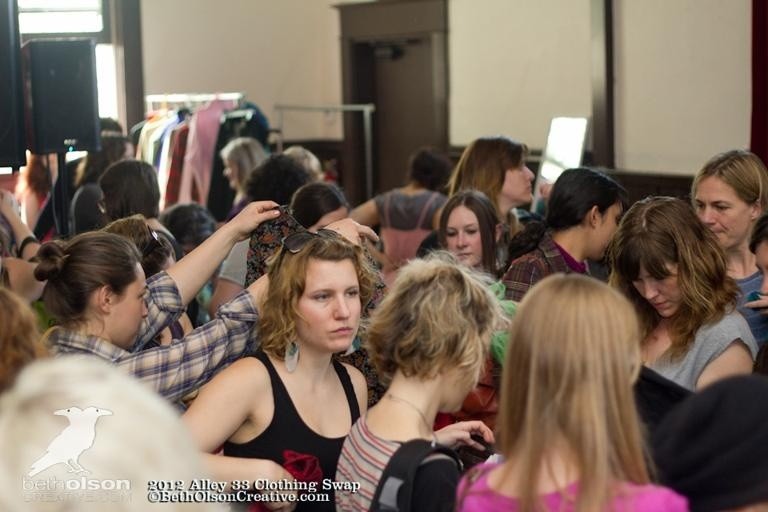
[651,375,768,511]
[183,229,368,512]
[454,271,689,512]
[35,201,380,415]
[332,253,514,512]
[1,119,767,392]
[0,285,231,512]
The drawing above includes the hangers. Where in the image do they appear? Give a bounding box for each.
[129,93,245,116]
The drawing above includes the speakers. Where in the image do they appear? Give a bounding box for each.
[19,38,102,153]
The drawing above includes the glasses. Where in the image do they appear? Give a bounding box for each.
[143,225,159,260]
[278,229,337,272]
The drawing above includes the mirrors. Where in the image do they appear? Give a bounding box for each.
[530,116,589,209]
